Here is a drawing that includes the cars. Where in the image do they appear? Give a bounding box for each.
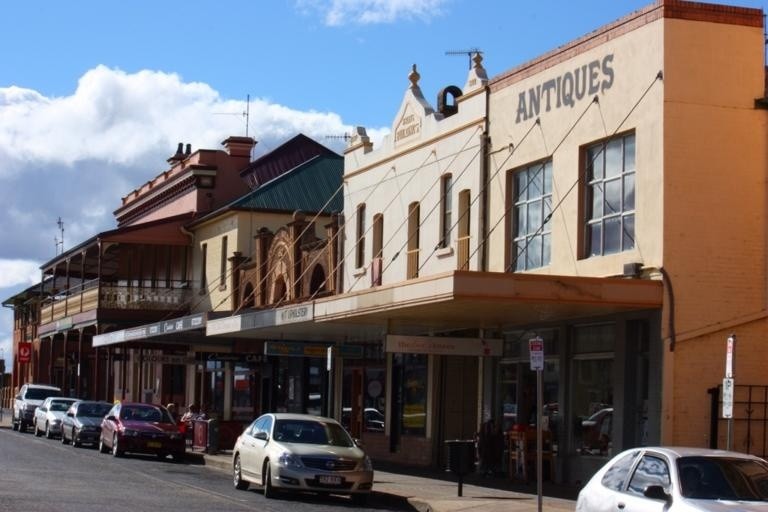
[33,397,188,461]
[231,411,373,502]
[576,446,768,512]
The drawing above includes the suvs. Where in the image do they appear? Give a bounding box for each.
[12,383,62,433]
